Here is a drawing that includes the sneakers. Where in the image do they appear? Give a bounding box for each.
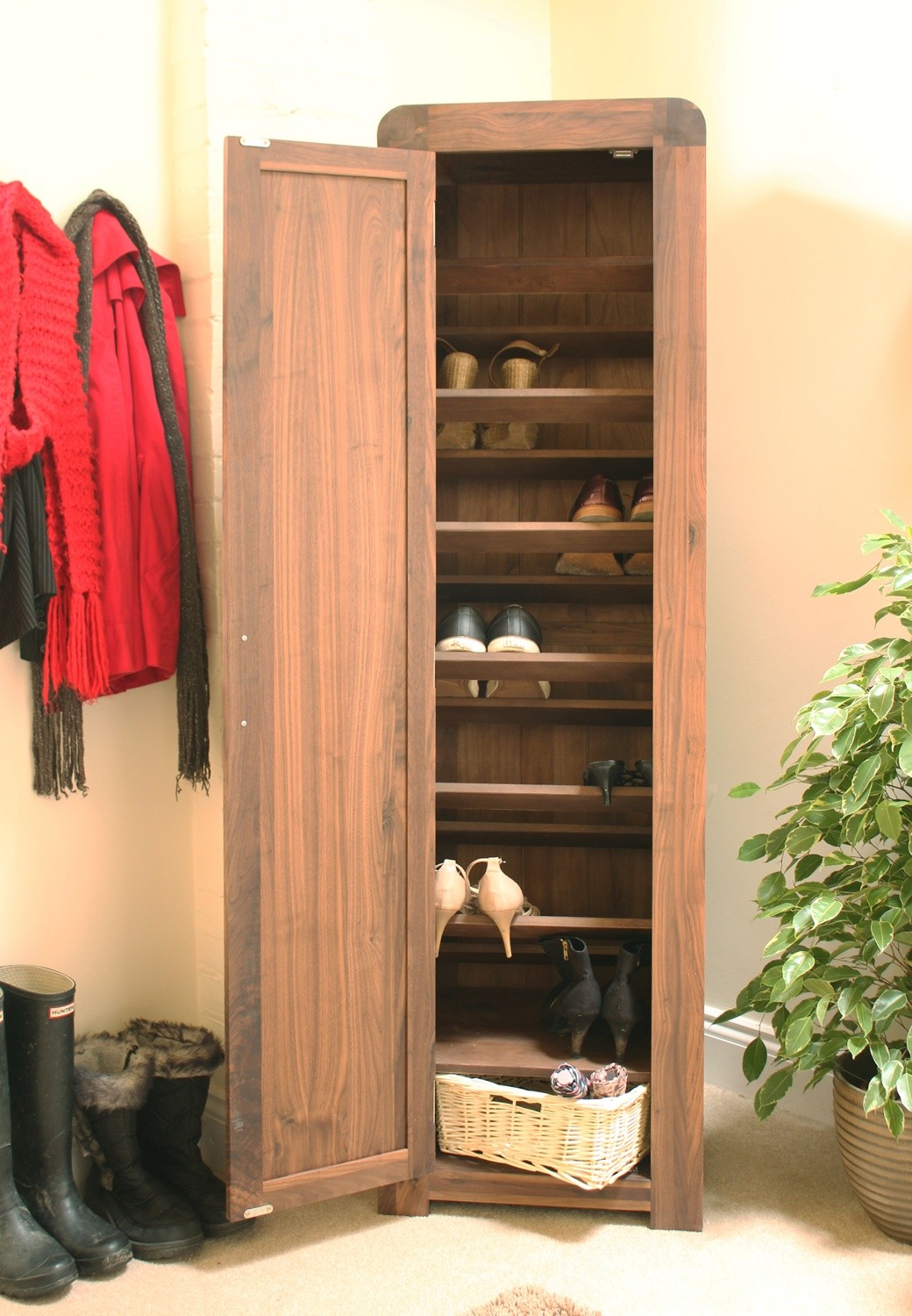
[435,599,551,698]
[562,472,653,576]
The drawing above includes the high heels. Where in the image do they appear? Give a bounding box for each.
[433,857,472,958]
[583,757,625,805]
[634,758,653,786]
[467,855,542,957]
[435,337,560,452]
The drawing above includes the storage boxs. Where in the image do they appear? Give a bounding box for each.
[437,1072,650,1191]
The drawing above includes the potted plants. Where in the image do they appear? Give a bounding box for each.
[707,505,912,1246]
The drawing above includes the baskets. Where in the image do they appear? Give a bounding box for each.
[435,1071,649,1189]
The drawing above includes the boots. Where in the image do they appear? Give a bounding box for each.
[598,939,648,1064]
[541,934,603,1058]
[0,962,135,1297]
[64,1015,257,1258]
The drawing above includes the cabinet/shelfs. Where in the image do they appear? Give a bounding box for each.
[228,96,707,1235]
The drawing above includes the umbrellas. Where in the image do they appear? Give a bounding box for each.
[589,1063,628,1100]
[550,1063,589,1100]
